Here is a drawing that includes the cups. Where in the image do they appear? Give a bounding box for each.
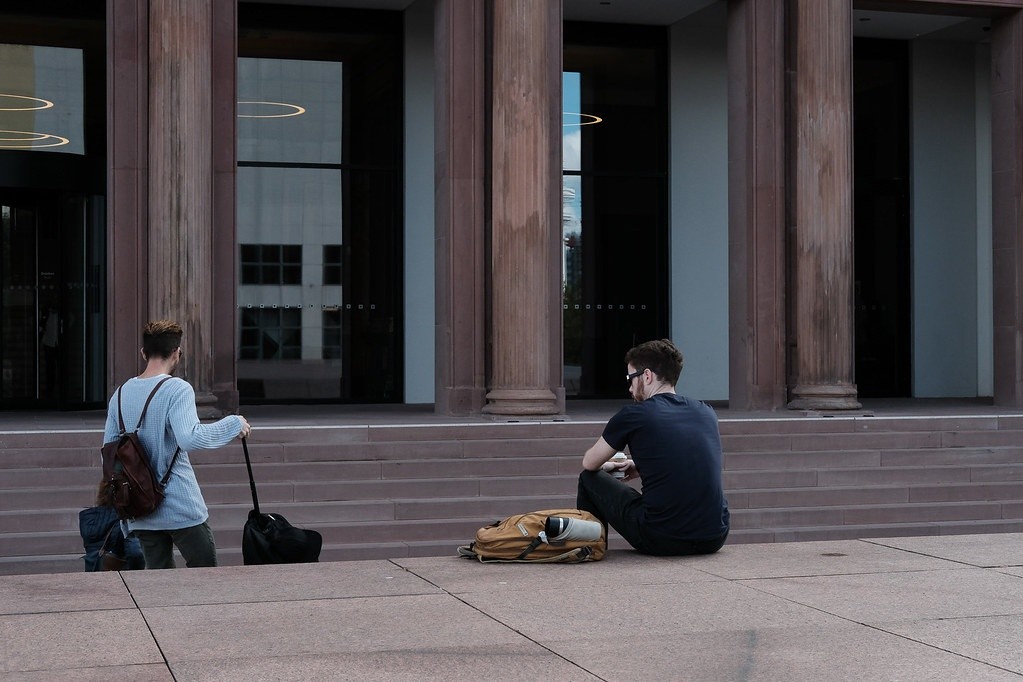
[608,453,627,478]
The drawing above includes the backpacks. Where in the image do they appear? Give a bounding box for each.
[78,504,146,572]
[94,375,181,525]
[456,508,606,563]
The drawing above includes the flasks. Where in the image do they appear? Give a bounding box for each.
[545,517,569,538]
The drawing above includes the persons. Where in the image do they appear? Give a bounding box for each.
[95,317,251,571]
[576,336,733,556]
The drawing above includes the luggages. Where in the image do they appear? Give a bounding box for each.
[239,435,323,566]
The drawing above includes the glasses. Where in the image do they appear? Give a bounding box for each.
[625,368,645,386]
